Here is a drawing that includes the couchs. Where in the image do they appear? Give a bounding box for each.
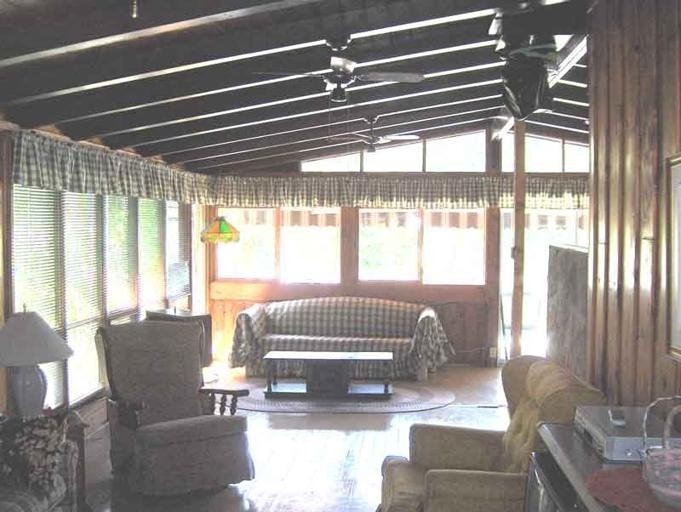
[246,297,435,382]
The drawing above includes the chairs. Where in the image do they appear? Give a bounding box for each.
[376,353,608,510]
[94,322,255,497]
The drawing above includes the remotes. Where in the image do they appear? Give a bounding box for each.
[608,409,626,426]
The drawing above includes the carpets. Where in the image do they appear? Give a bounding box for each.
[212,376,456,414]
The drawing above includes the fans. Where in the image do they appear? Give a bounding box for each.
[250,0,422,151]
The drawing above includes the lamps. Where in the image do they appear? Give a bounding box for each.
[198,167,240,246]
[2,312,74,422]
